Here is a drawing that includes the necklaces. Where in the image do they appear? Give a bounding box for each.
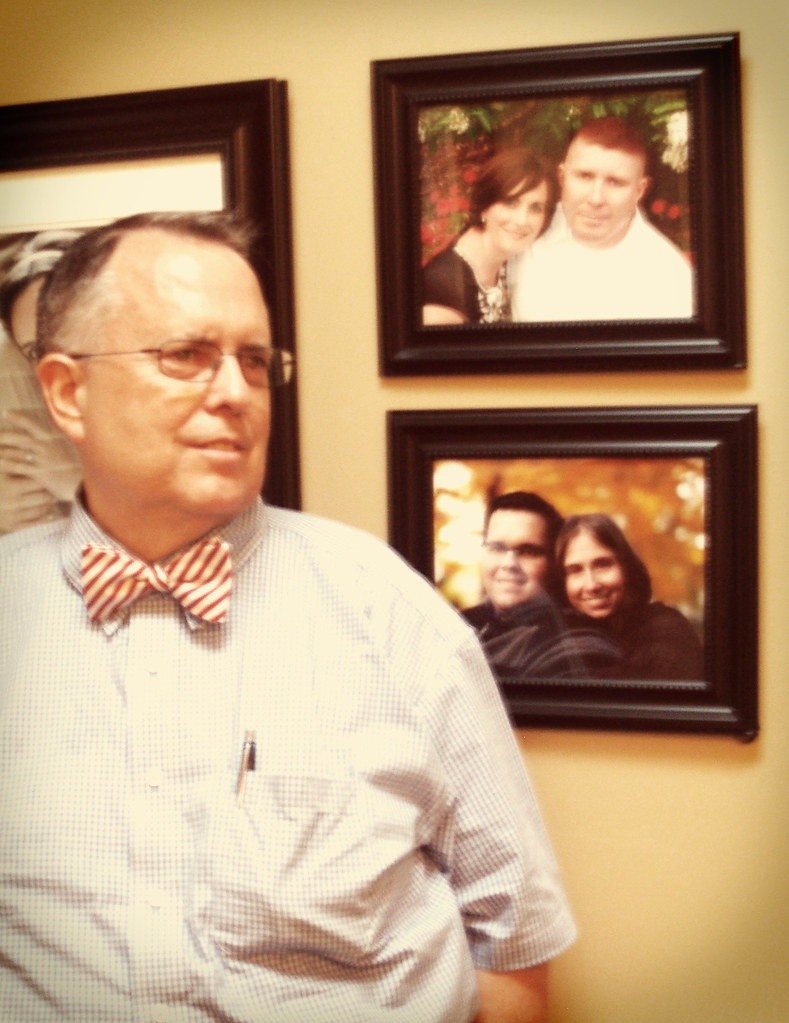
[422,147,556,327]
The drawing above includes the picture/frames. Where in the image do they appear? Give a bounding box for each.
[370,29,749,378]
[385,401,760,742]
[0,77,302,537]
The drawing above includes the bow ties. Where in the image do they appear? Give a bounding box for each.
[77,534,236,624]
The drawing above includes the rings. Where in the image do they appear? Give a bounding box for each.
[24,450,33,462]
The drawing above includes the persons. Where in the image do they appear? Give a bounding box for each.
[0,231,84,534]
[554,514,702,681]
[459,492,627,682]
[1,212,582,1023]
[505,113,695,319]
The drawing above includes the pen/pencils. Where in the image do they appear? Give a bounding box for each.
[236,730,255,792]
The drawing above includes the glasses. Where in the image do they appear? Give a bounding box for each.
[67,338,298,388]
[481,541,547,559]
